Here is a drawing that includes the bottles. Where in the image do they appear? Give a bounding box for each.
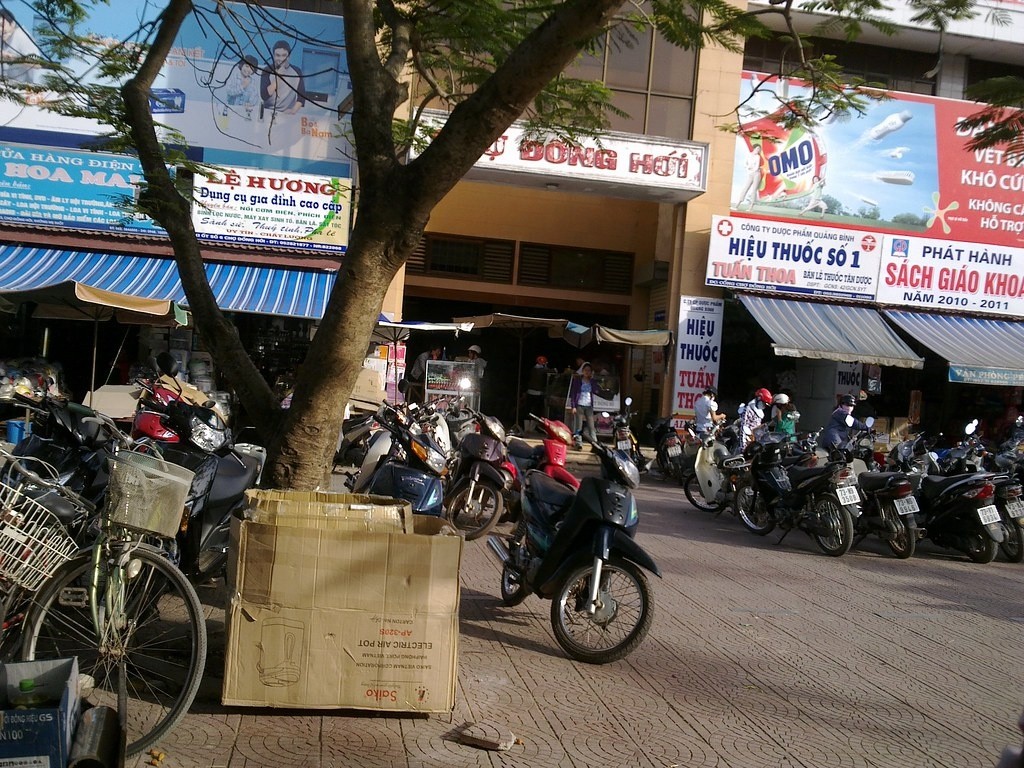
[221,111,228,130]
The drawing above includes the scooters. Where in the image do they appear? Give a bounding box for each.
[603,399,648,472]
[1,356,271,757]
[647,410,688,487]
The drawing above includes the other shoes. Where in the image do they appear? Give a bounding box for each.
[578,447,582,451]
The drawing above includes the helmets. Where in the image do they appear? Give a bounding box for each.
[468,345,481,353]
[840,394,857,406]
[755,388,772,406]
[537,355,547,365]
[773,393,790,404]
[703,386,717,396]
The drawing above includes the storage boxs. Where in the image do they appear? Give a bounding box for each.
[0,656,80,768]
[861,415,915,452]
[221,489,467,714]
[368,345,407,403]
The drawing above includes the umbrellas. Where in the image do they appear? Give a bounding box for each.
[0,280,190,409]
[369,317,471,407]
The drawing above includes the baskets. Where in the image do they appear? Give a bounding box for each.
[106,449,195,540]
[0,482,80,592]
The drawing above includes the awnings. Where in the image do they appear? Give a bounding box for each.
[738,295,925,369]
[885,309,1024,387]
[0,244,339,320]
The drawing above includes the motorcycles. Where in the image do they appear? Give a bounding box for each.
[689,406,1024,565]
[483,437,662,662]
[342,376,589,547]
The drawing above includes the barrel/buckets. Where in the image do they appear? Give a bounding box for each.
[6,420,32,444]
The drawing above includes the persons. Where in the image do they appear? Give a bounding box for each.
[260,40,305,114]
[0,9,40,85]
[464,345,487,378]
[823,393,874,451]
[570,363,600,450]
[575,355,585,367]
[410,344,442,383]
[730,143,762,212]
[694,386,726,437]
[739,388,797,447]
[526,355,556,420]
[226,55,258,107]
[800,175,828,218]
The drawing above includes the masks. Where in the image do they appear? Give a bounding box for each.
[756,401,765,409]
[711,397,715,401]
[842,405,854,415]
